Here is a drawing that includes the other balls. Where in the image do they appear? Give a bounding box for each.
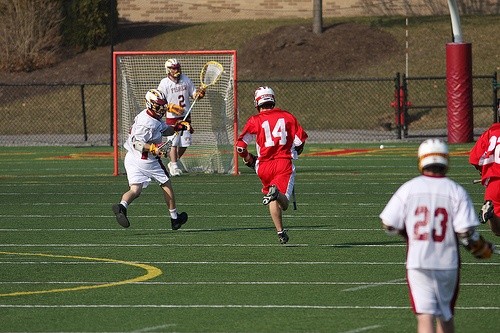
[380,144,384,149]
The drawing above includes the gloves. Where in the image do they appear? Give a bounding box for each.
[173,120,194,135]
[195,87,206,98]
[167,103,185,115]
[243,153,259,169]
[461,232,493,258]
[150,142,167,158]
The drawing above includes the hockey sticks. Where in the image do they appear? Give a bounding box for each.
[292,184,298,211]
[171,61,224,141]
[150,120,191,157]
[473,179,482,184]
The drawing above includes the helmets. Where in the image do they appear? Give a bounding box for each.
[418,139,449,174]
[145,89,168,118]
[253,86,275,107]
[165,59,181,77]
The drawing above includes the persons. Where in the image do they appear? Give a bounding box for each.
[236,85,308,243]
[468,104,500,237]
[378,138,494,333]
[158,58,206,176]
[112,90,194,231]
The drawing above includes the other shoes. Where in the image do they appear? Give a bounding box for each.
[478,200,494,223]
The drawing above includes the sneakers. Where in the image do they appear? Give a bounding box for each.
[171,212,188,230]
[262,184,278,205]
[112,204,130,228]
[168,162,180,176]
[278,230,289,244]
[177,160,186,172]
